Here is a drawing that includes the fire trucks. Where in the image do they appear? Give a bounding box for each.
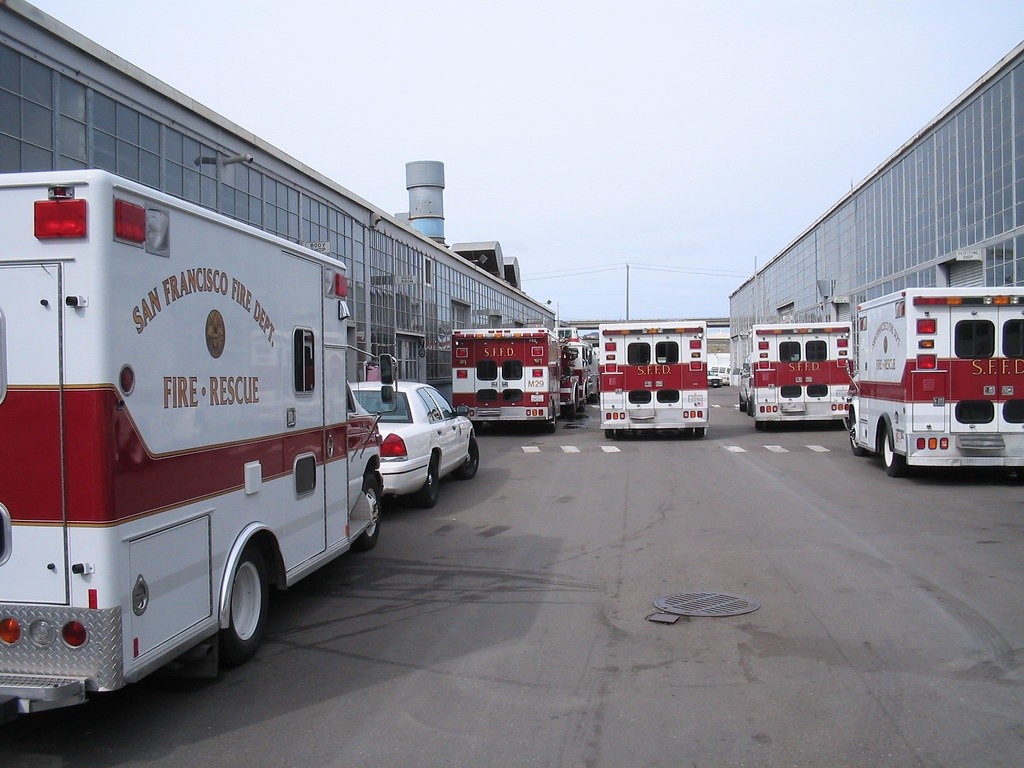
[737,322,851,430]
[0,169,400,713]
[598,321,708,438]
[843,284,1024,478]
[451,325,601,432]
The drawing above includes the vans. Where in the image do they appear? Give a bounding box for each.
[710,365,732,386]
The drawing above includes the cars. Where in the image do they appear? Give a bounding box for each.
[346,380,478,511]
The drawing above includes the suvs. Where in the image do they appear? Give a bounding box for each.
[706,371,723,387]
